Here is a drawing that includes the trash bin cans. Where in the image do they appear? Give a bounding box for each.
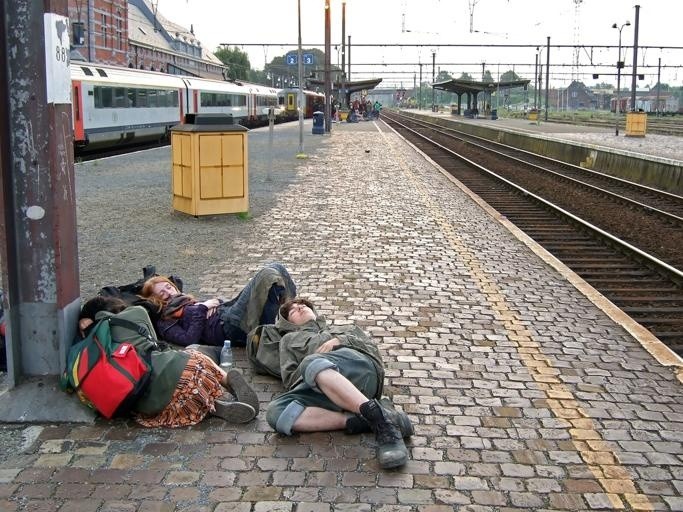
[312,111,324,134]
[491,109,498,120]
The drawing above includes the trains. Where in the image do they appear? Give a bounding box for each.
[282,87,325,120]
[401,96,418,109]
[609,95,680,115]
[69,61,286,152]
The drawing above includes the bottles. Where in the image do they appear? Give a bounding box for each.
[219,340,233,366]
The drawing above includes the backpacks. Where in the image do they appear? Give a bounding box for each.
[60,317,162,418]
[97,264,184,319]
[247,323,283,380]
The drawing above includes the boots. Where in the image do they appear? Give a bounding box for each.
[345,398,414,438]
[225,370,258,417]
[211,392,256,424]
[359,398,408,469]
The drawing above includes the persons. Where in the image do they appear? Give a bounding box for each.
[78,297,259,423]
[143,263,295,346]
[352,97,382,121]
[265,299,414,468]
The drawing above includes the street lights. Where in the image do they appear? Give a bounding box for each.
[335,45,346,102]
[536,47,545,111]
[480,61,487,110]
[612,20,630,134]
